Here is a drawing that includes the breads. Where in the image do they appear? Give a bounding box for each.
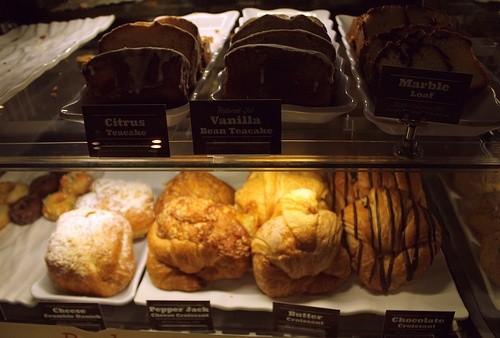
[453,168,500,290]
[80,6,500,122]
[44,171,443,297]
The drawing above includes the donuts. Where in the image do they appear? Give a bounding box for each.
[0,170,91,231]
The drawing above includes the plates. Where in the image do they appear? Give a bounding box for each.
[336,14,500,138]
[436,167,500,312]
[0,15,116,107]
[133,170,471,320]
[59,9,240,127]
[209,6,357,124]
[30,171,180,306]
[0,168,102,308]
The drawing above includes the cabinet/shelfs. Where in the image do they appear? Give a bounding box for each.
[0,45,500,338]
